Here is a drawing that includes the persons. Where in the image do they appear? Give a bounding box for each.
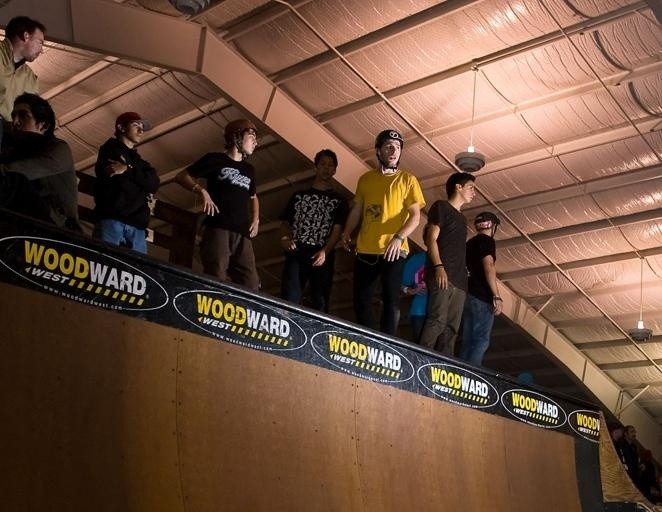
[457,211,504,368]
[419,171,476,357]
[278,148,349,313]
[1,93,84,234]
[607,421,662,503]
[398,224,429,343]
[93,111,160,253]
[0,15,47,122]
[340,128,427,336]
[174,118,261,291]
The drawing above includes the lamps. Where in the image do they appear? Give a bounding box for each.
[627,257,654,343]
[454,63,488,170]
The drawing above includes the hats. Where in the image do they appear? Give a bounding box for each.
[114,111,152,130]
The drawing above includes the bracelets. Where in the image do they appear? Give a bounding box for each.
[280,235,290,241]
[493,297,501,300]
[433,264,445,268]
[192,183,198,192]
[393,233,403,241]
[403,287,409,295]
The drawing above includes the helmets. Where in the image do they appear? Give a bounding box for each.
[224,118,257,133]
[374,130,403,149]
[474,211,501,229]
